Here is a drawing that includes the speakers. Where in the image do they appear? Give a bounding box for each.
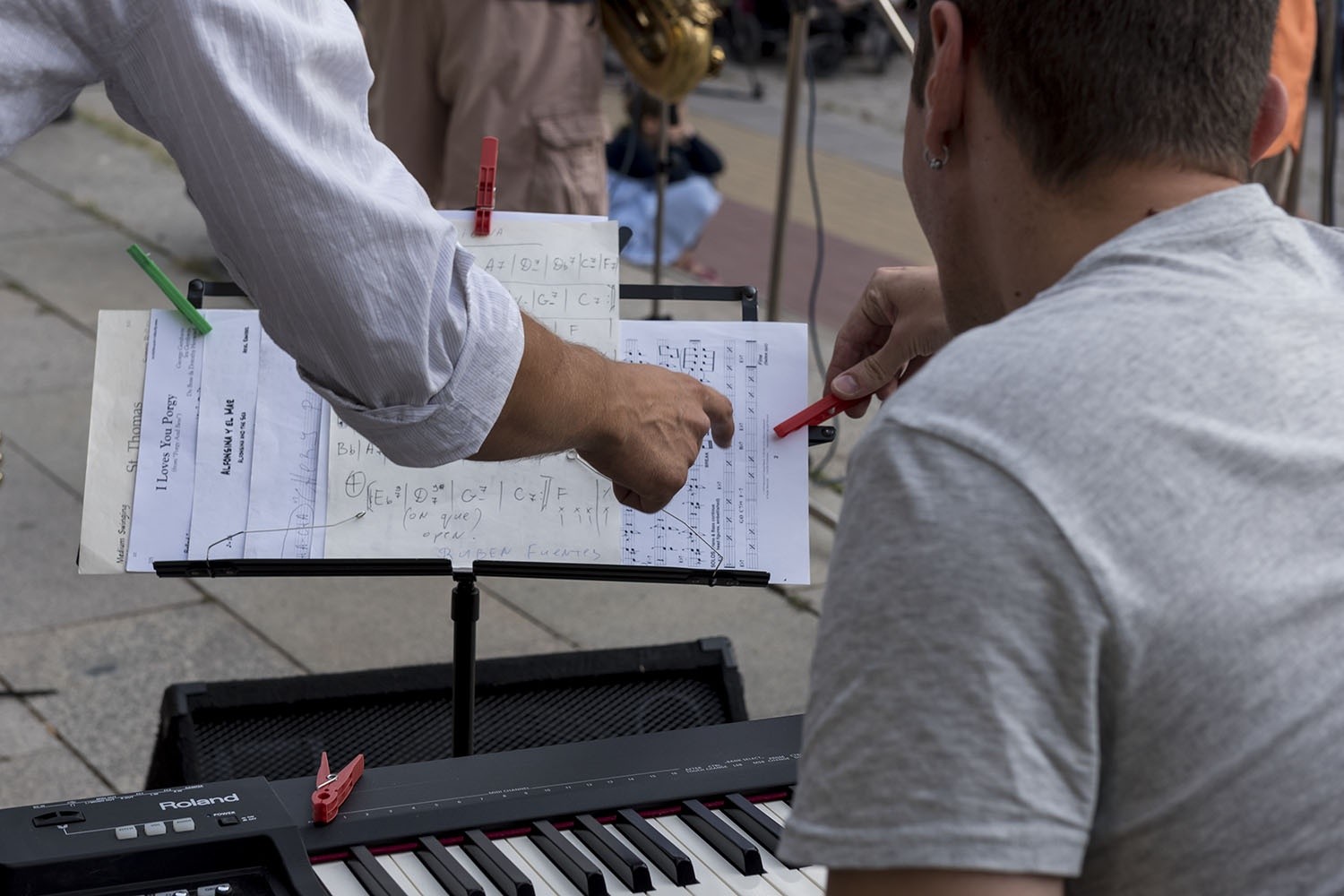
[143,634,751,791]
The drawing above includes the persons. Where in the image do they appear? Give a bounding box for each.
[776,0,1343,896]
[1,0,735,518]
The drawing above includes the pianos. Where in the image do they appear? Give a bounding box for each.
[0,711,826,896]
